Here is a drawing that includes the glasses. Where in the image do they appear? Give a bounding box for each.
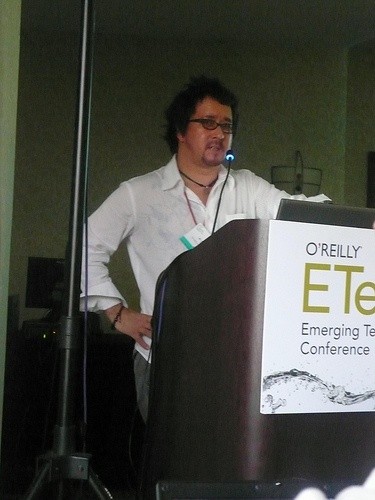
[188,118,235,134]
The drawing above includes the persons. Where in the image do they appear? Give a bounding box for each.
[79,75,334,426]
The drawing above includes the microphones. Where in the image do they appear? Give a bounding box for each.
[211,150,235,235]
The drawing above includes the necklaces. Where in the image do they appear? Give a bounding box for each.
[178,169,219,195]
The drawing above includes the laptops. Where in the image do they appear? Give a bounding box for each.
[276,199,375,229]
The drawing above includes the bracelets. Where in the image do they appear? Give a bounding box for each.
[110,304,127,330]
